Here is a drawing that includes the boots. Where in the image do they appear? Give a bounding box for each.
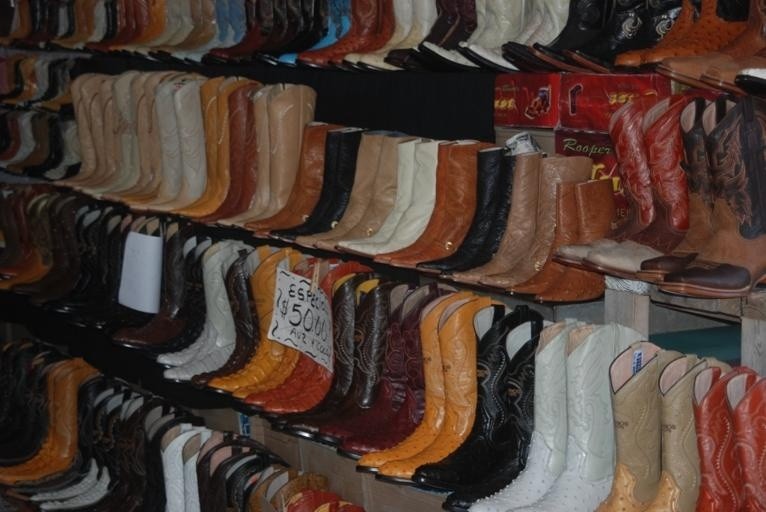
[0,0,766,107]
[551,94,766,301]
[1,179,763,512]
[0,70,619,306]
